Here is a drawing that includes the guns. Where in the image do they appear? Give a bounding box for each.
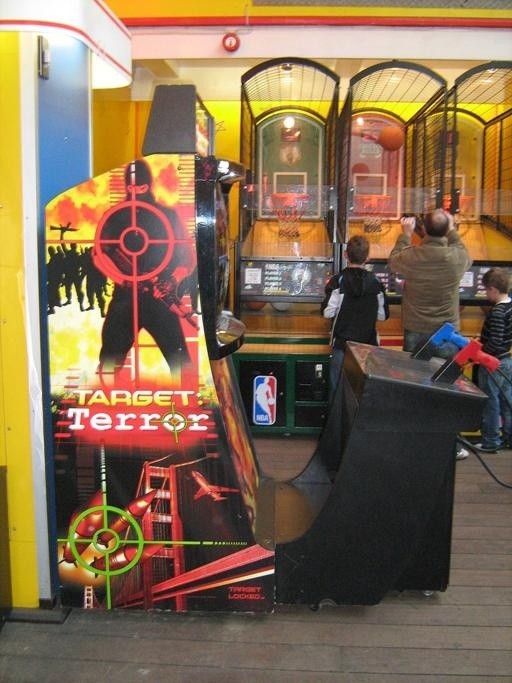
[108,247,196,326]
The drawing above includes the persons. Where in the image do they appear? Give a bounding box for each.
[320,235,390,407]
[473,267,511,454]
[389,207,473,459]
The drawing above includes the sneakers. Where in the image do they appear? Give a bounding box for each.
[475,442,498,452]
[495,438,512,449]
[455,448,469,459]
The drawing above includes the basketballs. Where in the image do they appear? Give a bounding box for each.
[270,302,292,311]
[378,126,404,151]
[246,301,266,310]
